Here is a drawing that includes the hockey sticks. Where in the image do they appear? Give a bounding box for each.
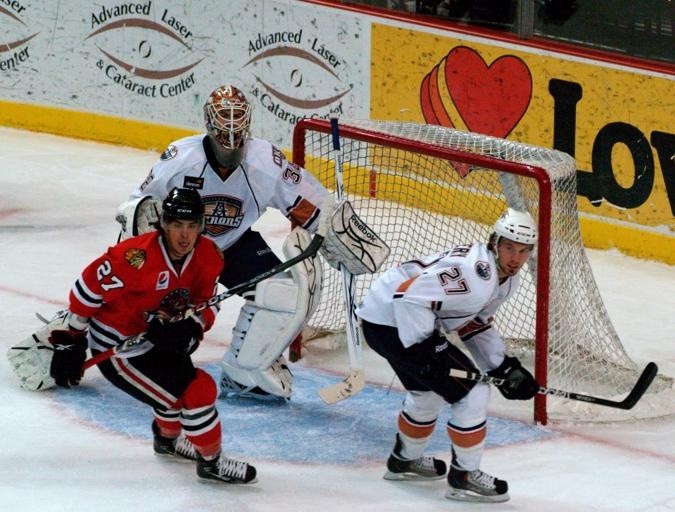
[450,362,658,410]
[318,114,366,405]
[81,206,331,369]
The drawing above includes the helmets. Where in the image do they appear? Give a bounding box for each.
[201,83,252,151]
[158,185,207,228]
[488,206,537,271]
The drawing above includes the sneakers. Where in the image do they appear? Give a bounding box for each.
[219,355,294,402]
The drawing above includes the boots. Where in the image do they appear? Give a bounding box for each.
[193,451,256,484]
[385,451,448,479]
[447,466,506,496]
[151,435,201,461]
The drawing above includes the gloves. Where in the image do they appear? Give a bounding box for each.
[46,328,86,389]
[493,358,540,401]
[148,312,205,354]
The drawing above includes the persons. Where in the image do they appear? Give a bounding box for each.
[48,187,259,484]
[355,207,539,495]
[6,86,391,396]
